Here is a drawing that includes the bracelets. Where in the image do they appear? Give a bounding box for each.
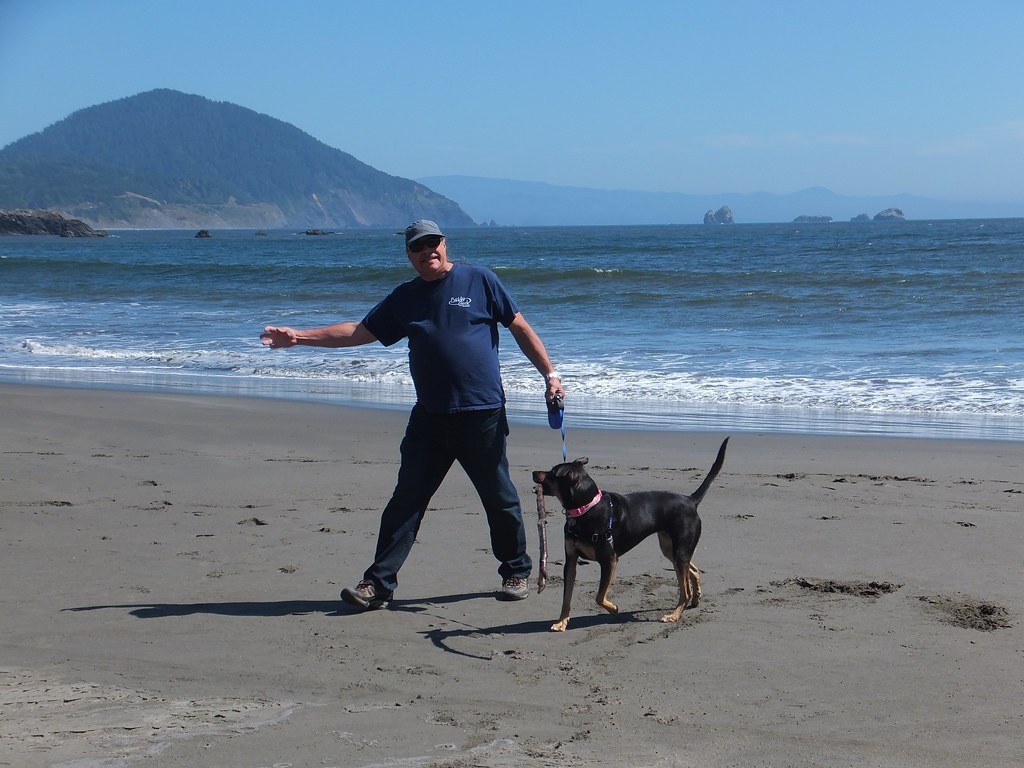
[545,372,561,383]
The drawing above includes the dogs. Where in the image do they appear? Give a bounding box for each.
[531,436,729,633]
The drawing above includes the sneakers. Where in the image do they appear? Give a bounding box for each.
[502,576,530,599]
[340,578,394,609]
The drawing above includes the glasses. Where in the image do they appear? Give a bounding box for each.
[408,236,442,253]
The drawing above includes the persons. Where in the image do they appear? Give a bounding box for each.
[259,220,566,608]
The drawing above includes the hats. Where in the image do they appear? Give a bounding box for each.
[405,219,445,248]
